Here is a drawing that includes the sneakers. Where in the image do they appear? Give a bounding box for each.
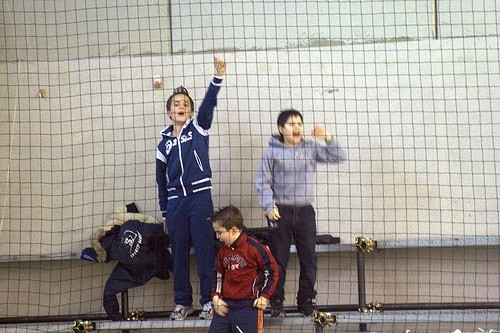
[270,297,286,318]
[170,304,194,321]
[297,297,320,318]
[199,301,215,320]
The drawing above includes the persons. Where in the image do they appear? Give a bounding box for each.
[208,206,279,333]
[155,57,226,321]
[255,109,346,317]
